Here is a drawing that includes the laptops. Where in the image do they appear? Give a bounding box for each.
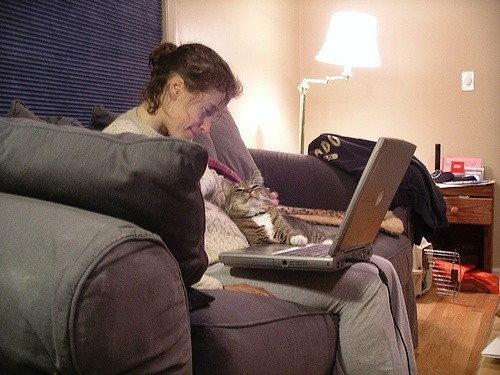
[435,143,477,182]
[219,137,417,273]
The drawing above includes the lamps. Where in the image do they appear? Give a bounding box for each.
[295,8,383,154]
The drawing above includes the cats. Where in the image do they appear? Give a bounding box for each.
[222,170,336,247]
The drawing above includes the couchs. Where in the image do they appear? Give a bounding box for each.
[0,146,419,375]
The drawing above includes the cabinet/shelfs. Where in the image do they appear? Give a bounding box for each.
[432,179,495,273]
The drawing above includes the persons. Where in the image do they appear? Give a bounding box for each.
[101,42,417,375]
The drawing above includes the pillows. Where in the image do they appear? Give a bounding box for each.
[1,114,216,314]
[192,102,266,185]
[89,101,123,129]
[6,96,85,127]
